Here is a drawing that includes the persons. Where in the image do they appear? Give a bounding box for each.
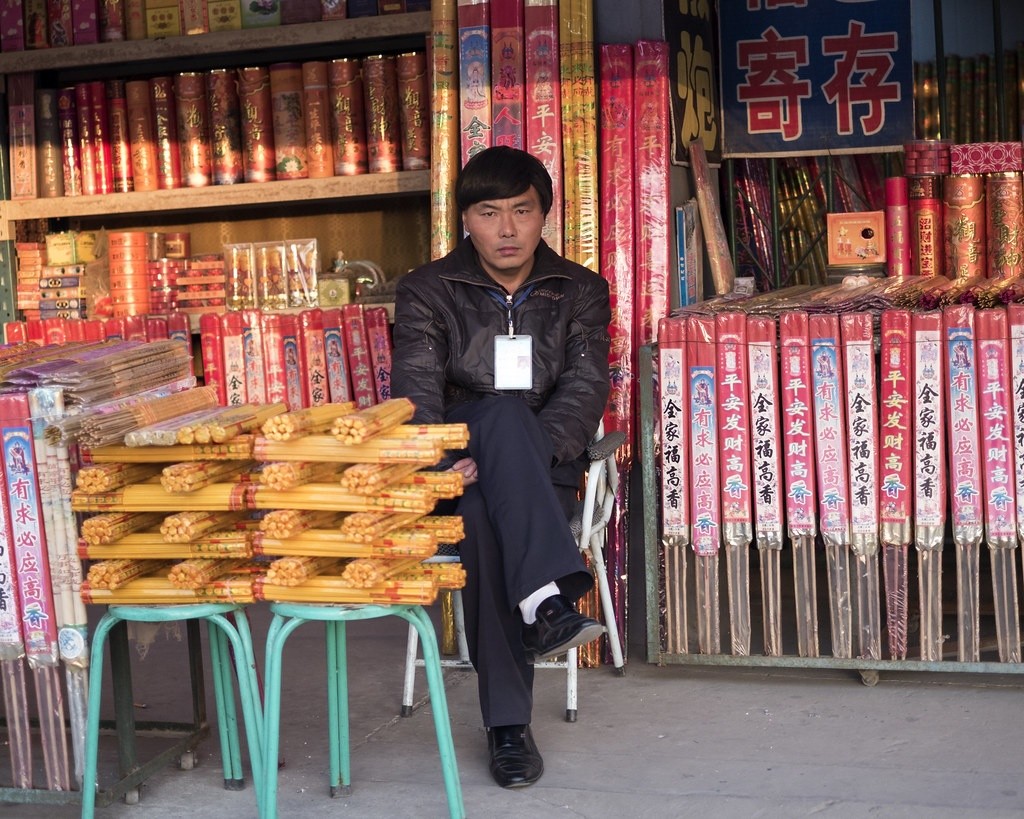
[388,144,611,789]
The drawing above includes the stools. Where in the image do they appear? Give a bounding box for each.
[80,602,262,819]
[261,602,466,819]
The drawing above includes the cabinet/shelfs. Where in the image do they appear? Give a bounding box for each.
[0,10,434,346]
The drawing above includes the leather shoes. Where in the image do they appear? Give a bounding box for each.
[486,724,545,788]
[515,594,603,665]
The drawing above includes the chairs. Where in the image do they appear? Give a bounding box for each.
[402,418,625,723]
